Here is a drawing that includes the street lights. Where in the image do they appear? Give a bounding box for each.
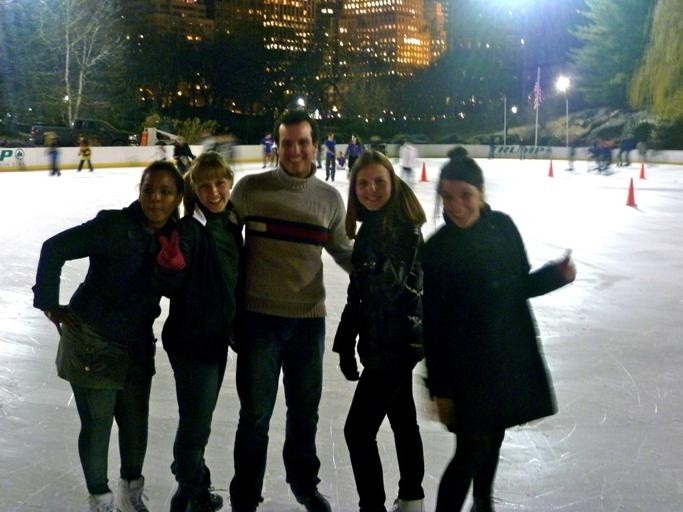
[556,74,572,147]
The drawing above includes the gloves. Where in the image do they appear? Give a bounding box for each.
[337,352,360,382]
[156,231,185,270]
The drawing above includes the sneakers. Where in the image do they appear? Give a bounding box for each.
[394,497,423,510]
[88,491,116,511]
[290,481,331,510]
[168,479,222,510]
[118,475,148,511]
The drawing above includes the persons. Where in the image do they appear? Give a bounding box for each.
[323,131,337,181]
[332,150,426,511]
[261,132,272,168]
[273,142,280,169]
[158,151,239,512]
[316,143,322,169]
[173,136,195,175]
[228,110,356,512]
[421,147,577,512]
[77,135,94,173]
[342,133,364,171]
[31,160,183,512]
[587,137,637,174]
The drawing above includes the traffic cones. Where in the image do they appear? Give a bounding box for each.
[548,159,553,177]
[626,177,639,209]
[418,160,430,184]
[639,161,648,181]
